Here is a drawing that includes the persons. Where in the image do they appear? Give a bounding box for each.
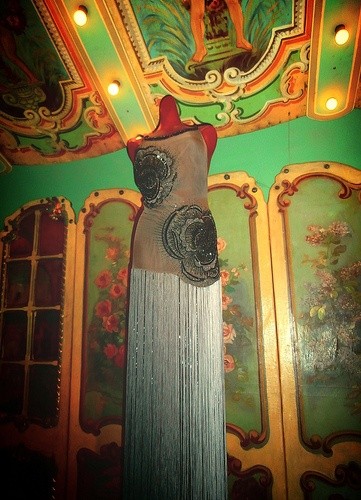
[126,92,230,499]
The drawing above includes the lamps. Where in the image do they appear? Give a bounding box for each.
[335,25,349,45]
[74,4,88,27]
[108,81,120,95]
